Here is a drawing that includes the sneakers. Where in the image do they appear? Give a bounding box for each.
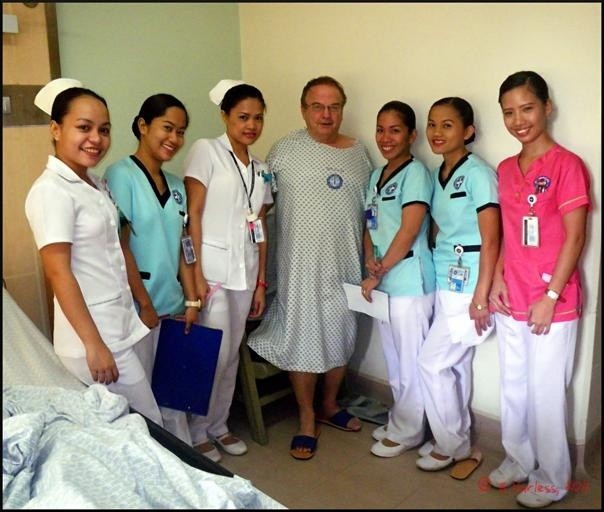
[487,463,524,489]
[516,482,554,508]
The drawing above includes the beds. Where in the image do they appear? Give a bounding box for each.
[1,283,289,509]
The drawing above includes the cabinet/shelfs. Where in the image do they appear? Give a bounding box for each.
[240,211,348,445]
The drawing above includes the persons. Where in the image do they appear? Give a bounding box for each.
[486,70,593,509]
[181,79,275,464]
[243,73,374,460]
[414,95,501,471]
[21,79,164,430]
[99,92,201,447]
[358,99,435,459]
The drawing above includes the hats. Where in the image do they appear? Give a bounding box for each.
[32,77,83,118]
[210,79,249,106]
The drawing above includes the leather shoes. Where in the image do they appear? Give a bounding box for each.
[372,423,388,441]
[213,431,248,456]
[415,453,455,471]
[418,439,435,457]
[192,438,221,464]
[370,436,412,460]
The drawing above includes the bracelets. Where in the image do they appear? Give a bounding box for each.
[183,298,202,312]
[256,280,269,290]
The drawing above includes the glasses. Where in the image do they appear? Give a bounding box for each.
[305,103,343,111]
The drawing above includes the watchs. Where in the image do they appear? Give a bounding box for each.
[544,287,559,301]
[470,297,488,311]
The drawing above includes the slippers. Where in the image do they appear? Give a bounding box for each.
[449,447,483,481]
[346,405,390,425]
[338,394,385,406]
[314,410,361,433]
[290,426,321,460]
